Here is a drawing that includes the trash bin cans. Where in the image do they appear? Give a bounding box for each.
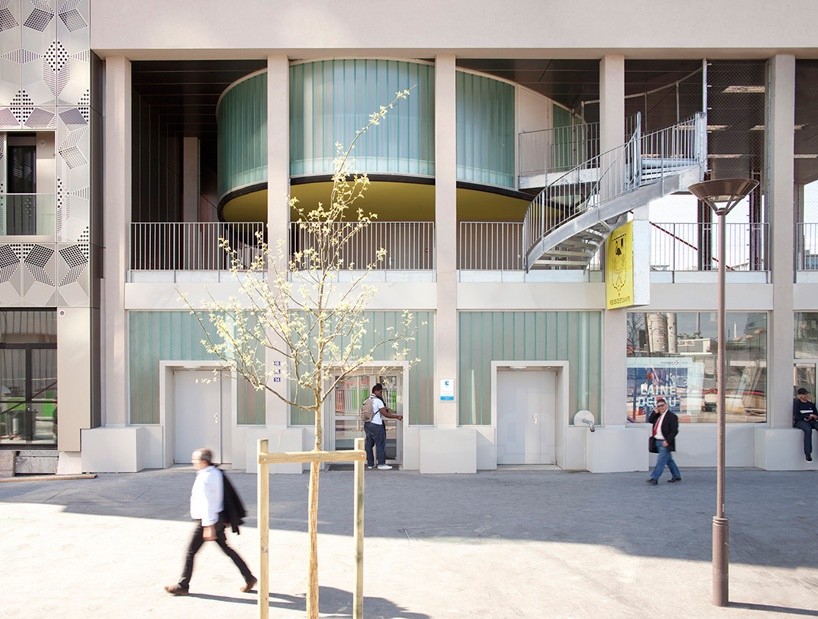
[7,410,38,440]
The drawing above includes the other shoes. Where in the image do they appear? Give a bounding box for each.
[368,466,372,469]
[806,453,812,461]
[165,585,188,595]
[667,477,681,483]
[647,479,658,485]
[240,577,257,592]
[378,464,392,470]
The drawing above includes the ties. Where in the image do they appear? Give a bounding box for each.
[654,414,662,436]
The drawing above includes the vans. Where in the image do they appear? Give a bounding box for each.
[726,360,817,416]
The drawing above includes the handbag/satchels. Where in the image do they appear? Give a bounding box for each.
[649,437,660,453]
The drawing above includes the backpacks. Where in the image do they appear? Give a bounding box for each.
[361,397,380,422]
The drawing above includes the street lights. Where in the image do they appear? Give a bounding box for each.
[686,177,762,609]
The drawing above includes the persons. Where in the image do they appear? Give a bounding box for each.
[645,399,682,485]
[361,383,403,470]
[793,388,818,464]
[165,448,258,596]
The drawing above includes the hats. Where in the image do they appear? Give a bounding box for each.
[797,388,810,394]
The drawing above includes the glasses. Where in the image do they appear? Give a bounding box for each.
[658,404,665,408]
